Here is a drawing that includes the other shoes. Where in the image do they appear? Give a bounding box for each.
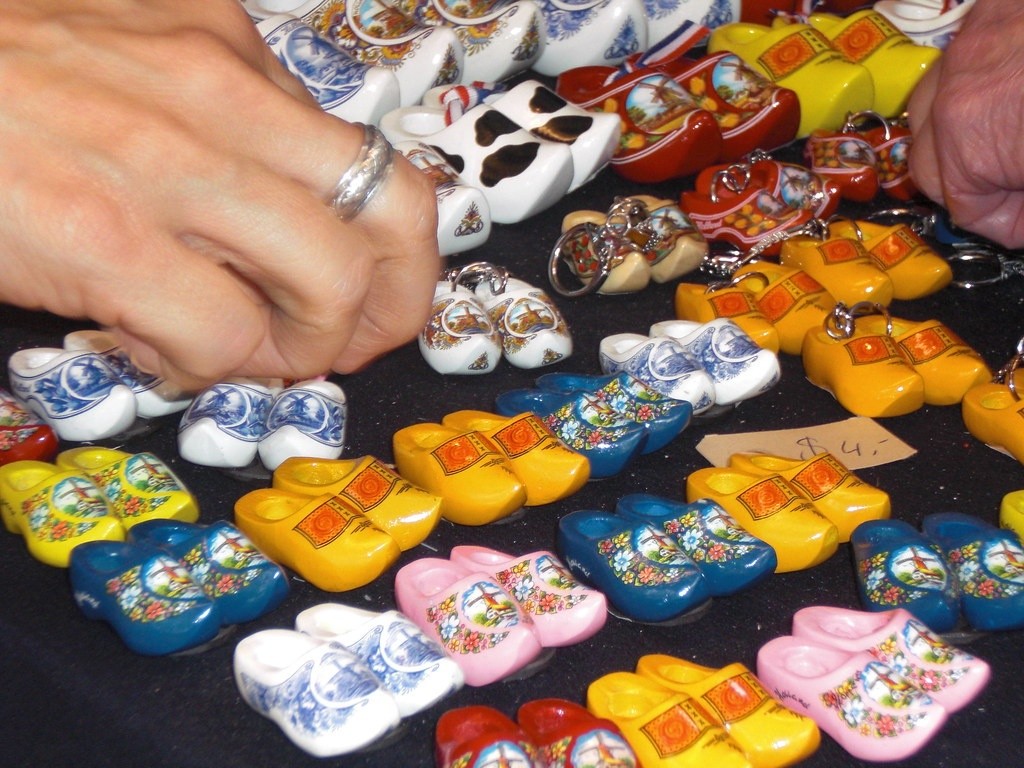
[1,0,1024,768]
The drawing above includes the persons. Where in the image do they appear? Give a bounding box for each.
[907,0,1024,251]
[0,0,438,395]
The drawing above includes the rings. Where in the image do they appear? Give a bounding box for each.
[328,120,396,224]
